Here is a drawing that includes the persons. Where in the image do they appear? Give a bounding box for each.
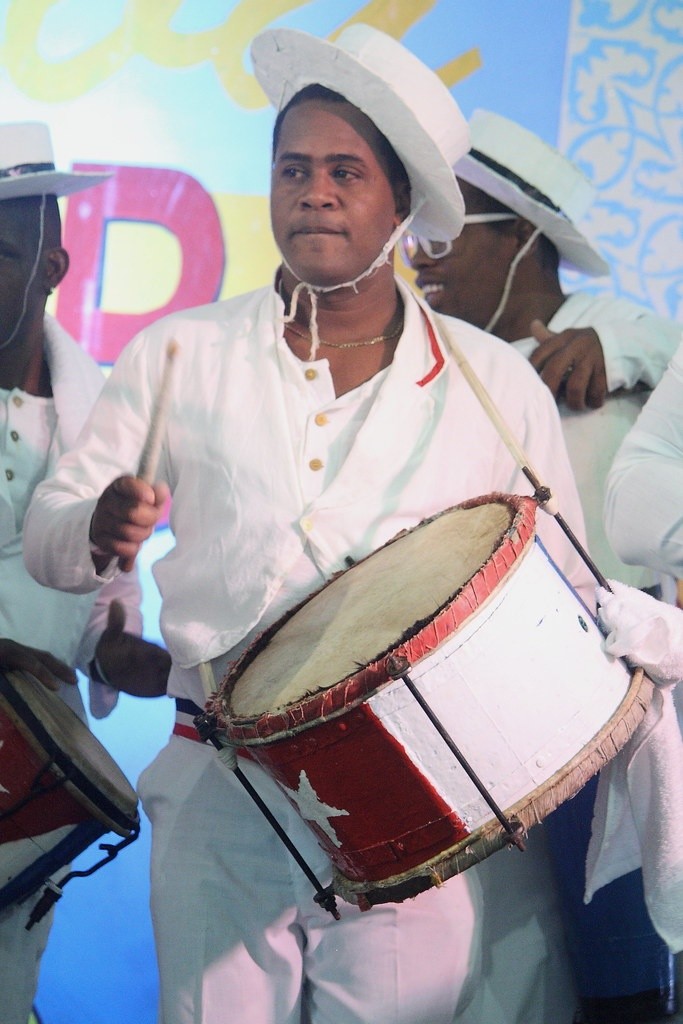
[22,20,597,1024]
[1,122,167,1022]
[402,113,682,1024]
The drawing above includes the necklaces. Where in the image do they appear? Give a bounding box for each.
[284,323,401,348]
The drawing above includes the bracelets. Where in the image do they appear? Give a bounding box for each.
[93,654,110,685]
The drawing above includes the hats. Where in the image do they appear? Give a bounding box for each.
[252,22,473,242]
[0,125,116,201]
[451,108,610,275]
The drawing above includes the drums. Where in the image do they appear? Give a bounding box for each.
[0,650,140,923]
[198,493,653,905]
